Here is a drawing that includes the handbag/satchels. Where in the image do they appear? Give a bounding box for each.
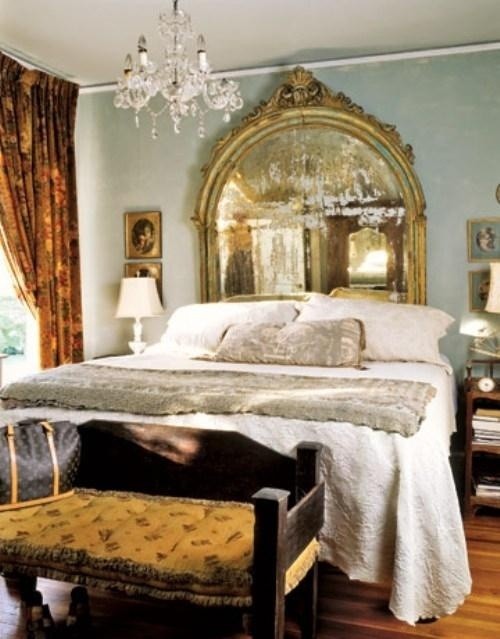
[1,420,81,511]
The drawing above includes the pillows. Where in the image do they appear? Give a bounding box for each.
[167,299,455,370]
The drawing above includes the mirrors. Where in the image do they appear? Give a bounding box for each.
[191,65,427,306]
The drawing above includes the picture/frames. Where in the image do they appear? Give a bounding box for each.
[124,210,163,259]
[470,271,490,313]
[124,261,163,307]
[467,219,499,263]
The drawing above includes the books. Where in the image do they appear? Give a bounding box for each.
[473,473,500,501]
[472,406,500,447]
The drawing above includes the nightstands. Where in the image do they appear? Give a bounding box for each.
[466,357,500,518]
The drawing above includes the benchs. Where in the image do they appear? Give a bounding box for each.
[0,419,324,639]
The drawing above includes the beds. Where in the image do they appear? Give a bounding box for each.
[1,291,474,629]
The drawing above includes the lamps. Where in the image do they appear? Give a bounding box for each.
[112,0,245,138]
[485,263,500,314]
[114,277,163,356]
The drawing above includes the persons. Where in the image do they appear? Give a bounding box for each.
[134,219,154,254]
